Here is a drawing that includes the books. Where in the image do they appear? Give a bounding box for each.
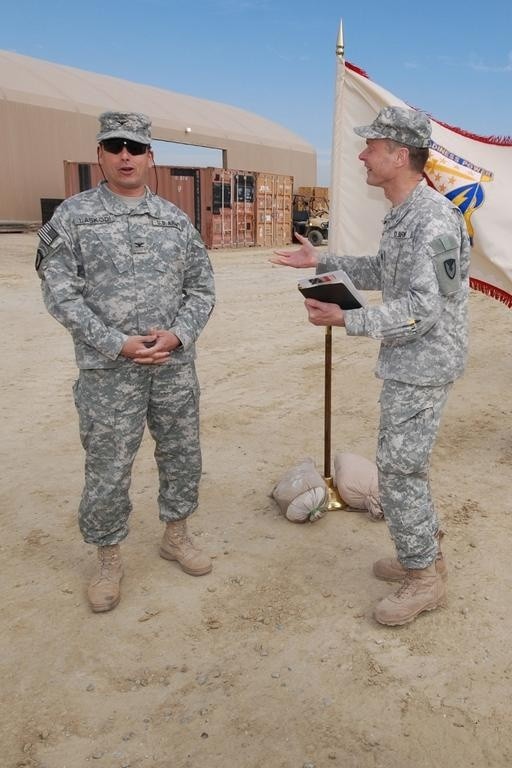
[292,267,368,314]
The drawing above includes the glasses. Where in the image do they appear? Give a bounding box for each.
[101,138,147,156]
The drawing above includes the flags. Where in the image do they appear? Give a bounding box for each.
[329,57,512,314]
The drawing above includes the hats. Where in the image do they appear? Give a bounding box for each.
[353,105,432,148]
[95,110,152,145]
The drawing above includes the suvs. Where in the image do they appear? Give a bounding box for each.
[292,194,330,246]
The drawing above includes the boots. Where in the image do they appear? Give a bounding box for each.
[373,557,448,584]
[374,561,446,626]
[86,544,124,613]
[158,520,213,577]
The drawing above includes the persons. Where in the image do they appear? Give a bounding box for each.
[263,103,473,630]
[34,109,218,612]
